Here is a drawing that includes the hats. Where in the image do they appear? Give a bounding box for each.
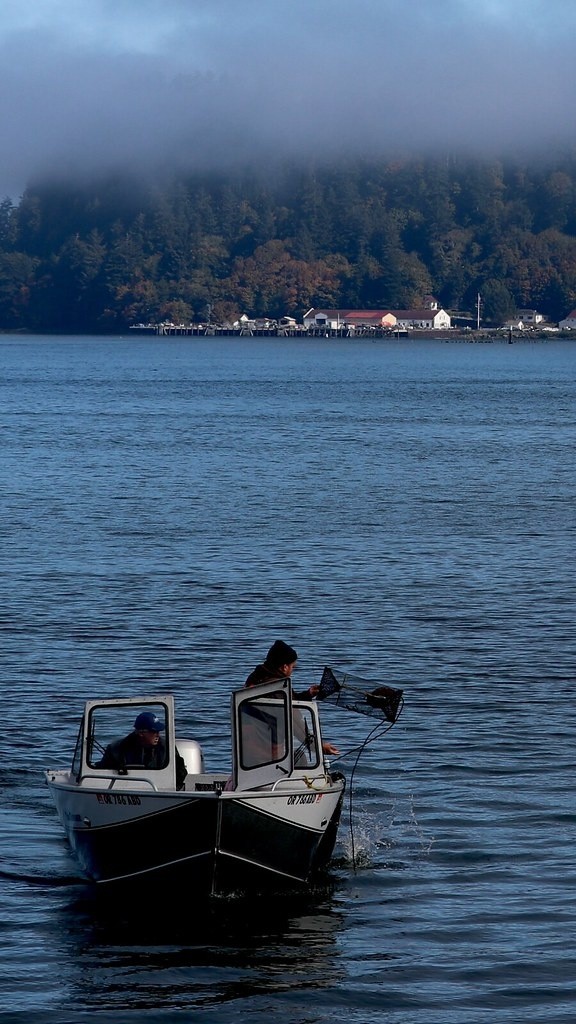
[267,640,298,668]
[135,712,164,731]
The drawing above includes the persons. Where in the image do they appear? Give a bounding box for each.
[98,712,187,792]
[225,639,341,769]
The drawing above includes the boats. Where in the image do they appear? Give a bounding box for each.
[45,680,349,906]
